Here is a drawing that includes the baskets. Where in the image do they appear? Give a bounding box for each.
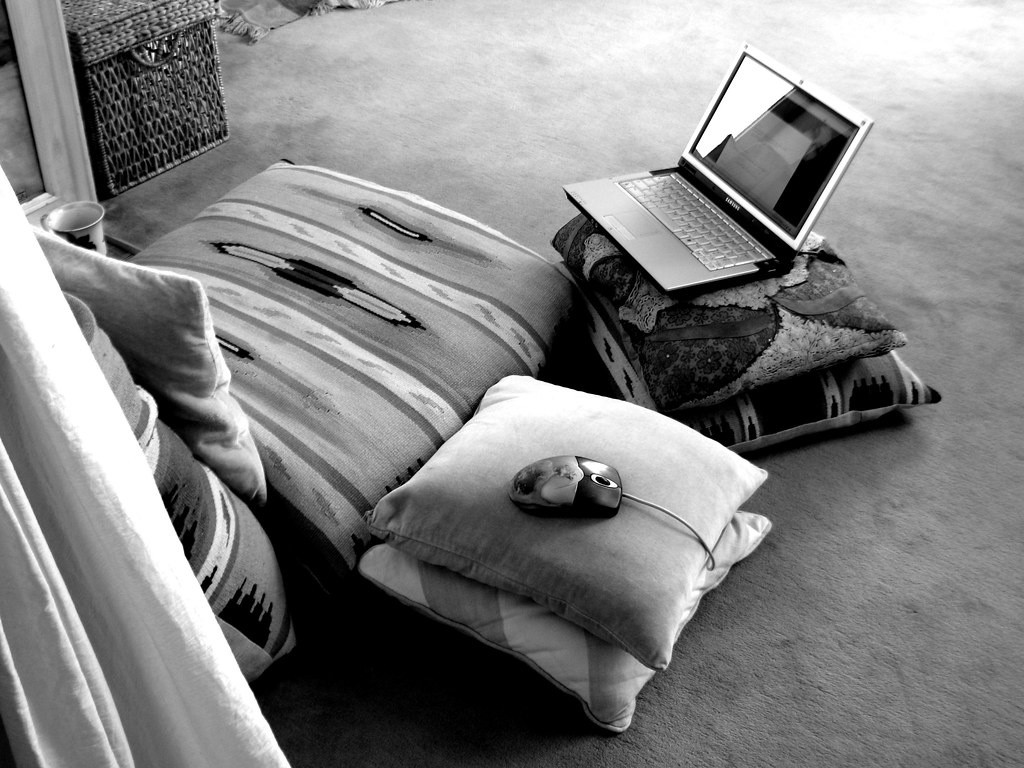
[61,0,230,197]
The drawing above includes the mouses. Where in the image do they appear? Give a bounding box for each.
[508,455,622,519]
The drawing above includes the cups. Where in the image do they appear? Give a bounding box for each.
[46,201,106,256]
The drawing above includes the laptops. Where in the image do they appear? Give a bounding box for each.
[562,41,874,295]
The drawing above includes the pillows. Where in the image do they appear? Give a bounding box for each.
[550,261,941,452]
[550,213,908,413]
[23,224,266,506]
[364,373,770,671]
[62,293,300,682]
[357,509,774,732]
